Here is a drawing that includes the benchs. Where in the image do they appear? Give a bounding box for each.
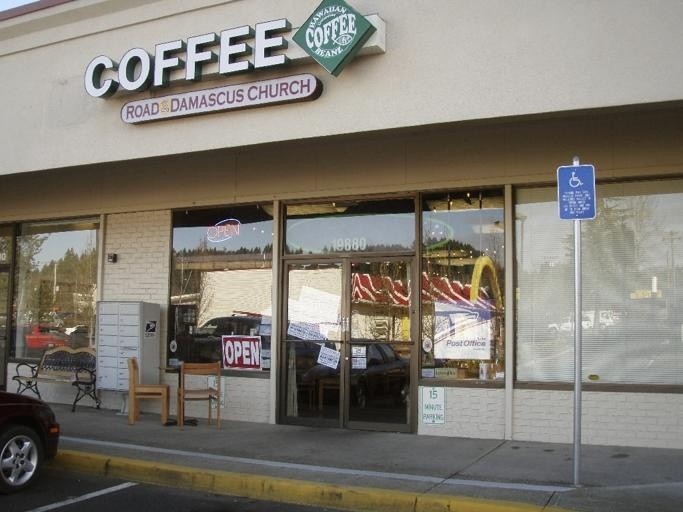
[12,346,96,399]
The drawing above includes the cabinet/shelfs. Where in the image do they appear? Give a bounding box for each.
[95,301,160,392]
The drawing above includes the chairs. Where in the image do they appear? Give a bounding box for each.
[71,368,100,412]
[127,357,169,425]
[177,362,222,431]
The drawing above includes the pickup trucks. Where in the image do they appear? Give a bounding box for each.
[549,316,588,332]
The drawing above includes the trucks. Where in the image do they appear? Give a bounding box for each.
[571,310,617,329]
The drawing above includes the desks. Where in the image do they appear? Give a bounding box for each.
[318,377,340,413]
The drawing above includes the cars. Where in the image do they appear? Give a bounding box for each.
[15,323,75,353]
[0,393,61,492]
[169,316,330,371]
[64,325,87,333]
[305,340,412,406]
